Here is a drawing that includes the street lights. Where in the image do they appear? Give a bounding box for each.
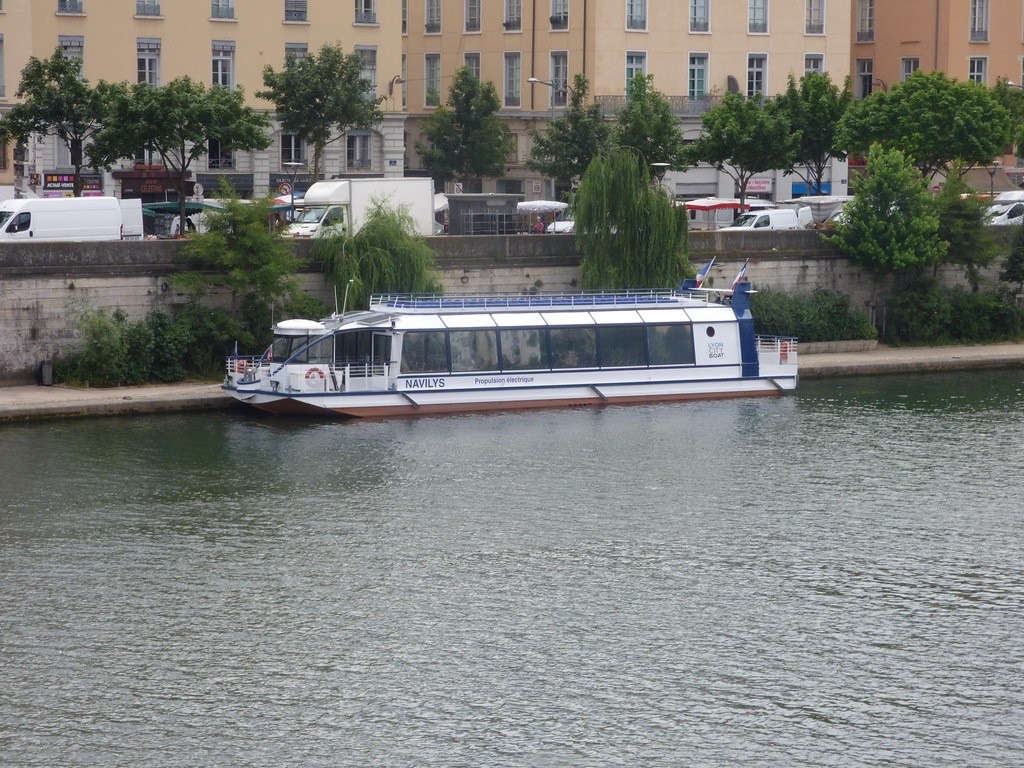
[528,77,556,201]
[282,162,305,221]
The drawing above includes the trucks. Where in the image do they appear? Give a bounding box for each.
[980,189,1024,225]
[280,177,436,240]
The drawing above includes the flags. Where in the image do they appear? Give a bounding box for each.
[695,261,712,287]
[733,260,747,291]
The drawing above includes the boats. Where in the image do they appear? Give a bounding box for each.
[220,287,797,420]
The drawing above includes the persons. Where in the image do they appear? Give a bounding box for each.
[534,217,544,234]
[185,217,197,235]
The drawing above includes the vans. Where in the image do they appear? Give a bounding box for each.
[547,205,618,235]
[0,197,145,240]
[718,206,814,228]
